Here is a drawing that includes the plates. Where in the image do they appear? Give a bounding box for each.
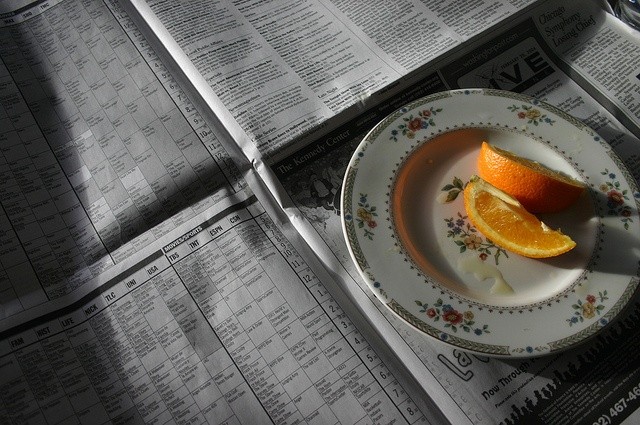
[340,88,639,361]
